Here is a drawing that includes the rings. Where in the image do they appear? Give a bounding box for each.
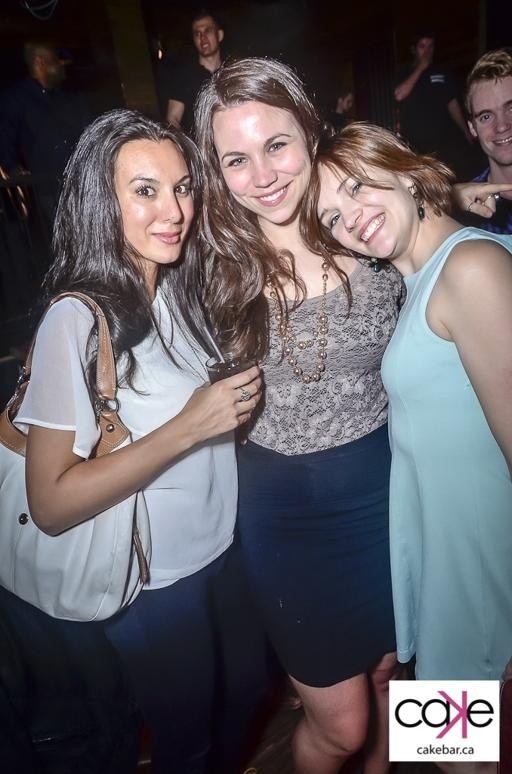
[467,200,474,211]
[494,193,499,199]
[238,386,251,401]
[484,193,492,202]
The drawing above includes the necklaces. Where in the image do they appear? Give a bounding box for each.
[265,259,330,383]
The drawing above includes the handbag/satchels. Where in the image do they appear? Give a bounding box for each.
[1,381,152,622]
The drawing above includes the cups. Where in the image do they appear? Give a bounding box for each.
[206,351,258,386]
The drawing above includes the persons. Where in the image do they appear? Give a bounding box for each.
[1,32,105,237]
[449,49,512,234]
[1,107,264,774]
[393,26,473,172]
[166,11,239,139]
[297,120,512,774]
[192,56,511,774]
